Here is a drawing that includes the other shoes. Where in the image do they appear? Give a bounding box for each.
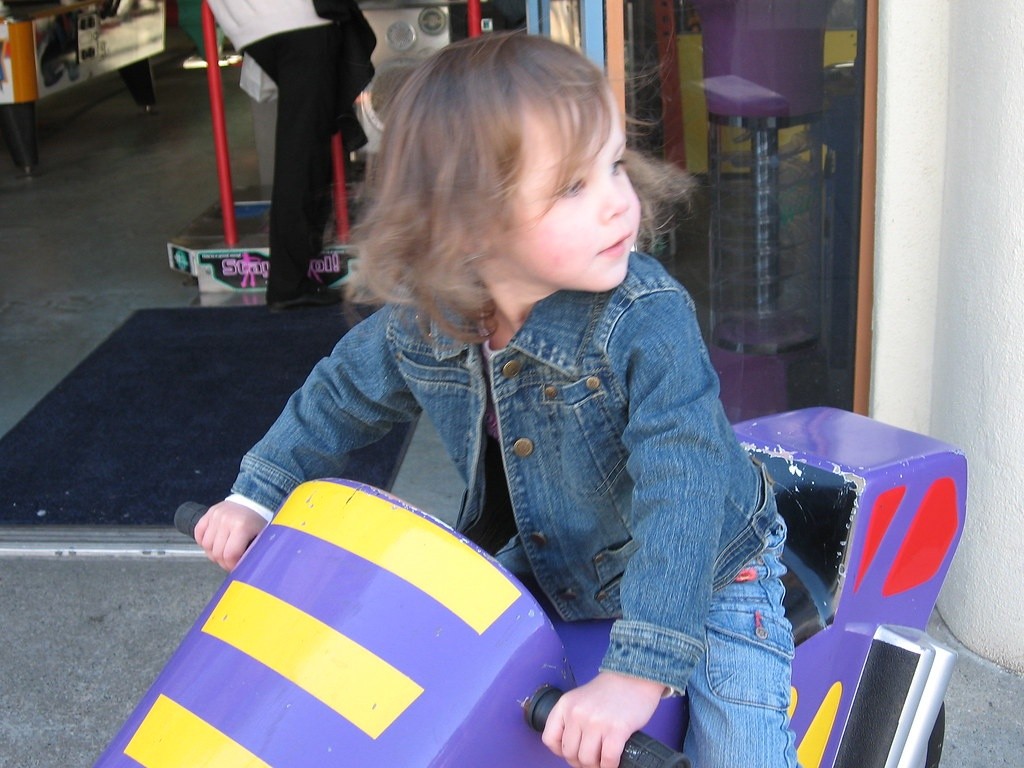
[265,274,345,312]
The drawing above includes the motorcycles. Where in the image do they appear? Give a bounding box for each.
[85,406,973,768]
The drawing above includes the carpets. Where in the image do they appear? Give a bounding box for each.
[1,305,520,555]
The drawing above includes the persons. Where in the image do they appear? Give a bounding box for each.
[208,1,377,312]
[194,30,806,767]
